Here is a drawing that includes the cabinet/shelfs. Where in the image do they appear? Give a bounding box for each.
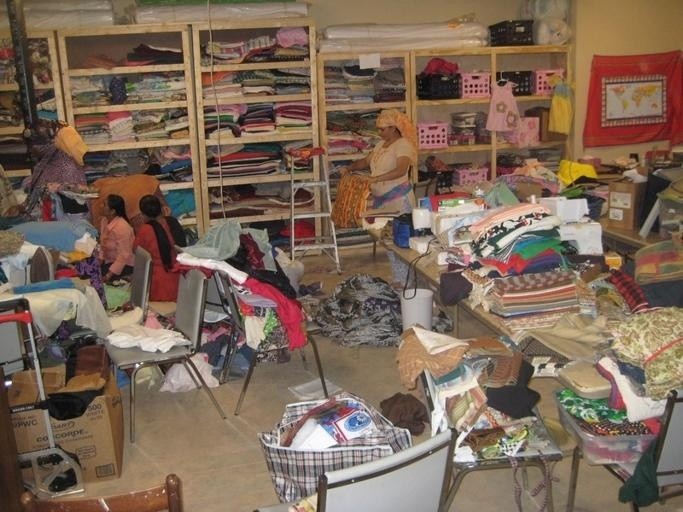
[1,16,575,245]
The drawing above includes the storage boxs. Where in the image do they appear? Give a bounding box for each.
[3,342,125,486]
[606,171,647,234]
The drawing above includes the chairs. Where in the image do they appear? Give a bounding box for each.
[417,357,565,510]
[18,473,184,512]
[57,246,155,385]
[212,269,330,418]
[105,266,226,444]
[177,246,232,385]
[242,224,278,272]
[567,390,681,509]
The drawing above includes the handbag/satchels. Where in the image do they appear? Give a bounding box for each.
[257,391,413,504]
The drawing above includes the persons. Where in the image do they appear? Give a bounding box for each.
[338,109,419,290]
[98,195,135,283]
[133,194,187,301]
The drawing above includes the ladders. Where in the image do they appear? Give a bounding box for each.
[287,146,342,275]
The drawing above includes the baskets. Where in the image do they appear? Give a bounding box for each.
[458,71,490,99]
[488,19,534,46]
[496,71,532,96]
[416,123,449,150]
[415,72,461,99]
[451,168,488,186]
[530,69,563,96]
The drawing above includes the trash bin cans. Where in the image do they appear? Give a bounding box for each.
[399,288,434,333]
